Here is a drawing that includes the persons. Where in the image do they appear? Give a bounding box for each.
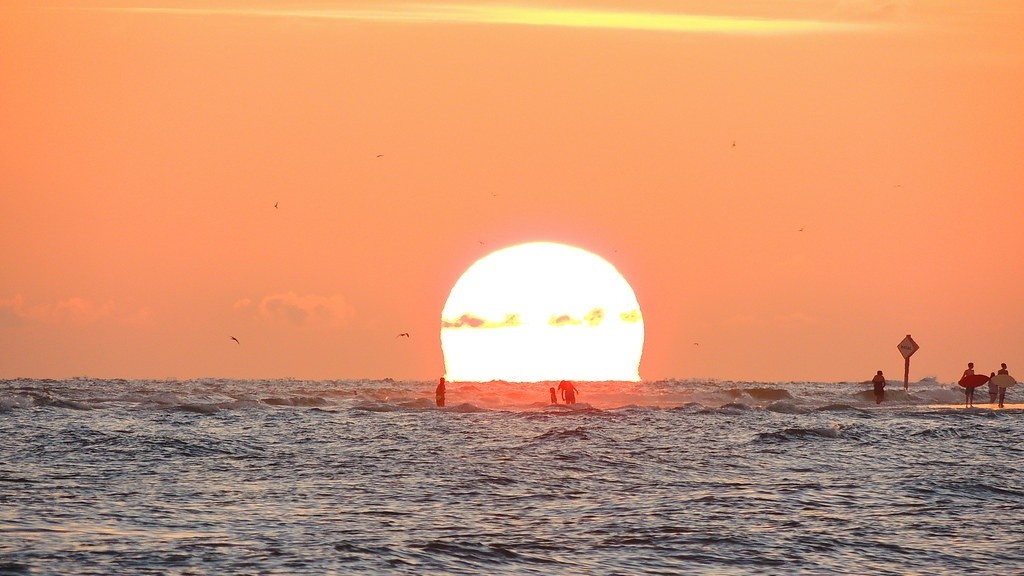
[958,362,975,407]
[558,380,578,404]
[872,370,886,404]
[988,372,998,404]
[549,387,557,403]
[436,378,446,407]
[998,363,1009,408]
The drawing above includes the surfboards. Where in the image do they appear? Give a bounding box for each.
[991,374,1015,387]
[958,374,990,388]
